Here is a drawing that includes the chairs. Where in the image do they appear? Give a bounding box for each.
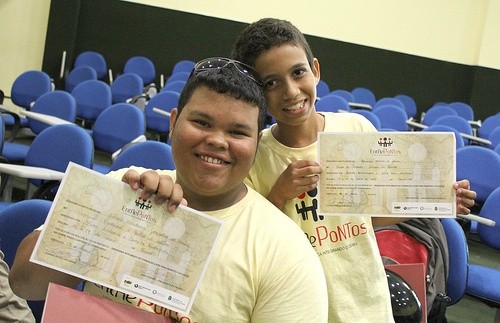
[0,51,500,323]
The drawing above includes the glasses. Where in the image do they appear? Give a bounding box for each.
[187,56,266,88]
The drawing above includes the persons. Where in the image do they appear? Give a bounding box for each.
[8,56,328,323]
[229,17,477,323]
[0,249,37,323]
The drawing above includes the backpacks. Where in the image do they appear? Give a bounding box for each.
[372,217,450,323]
